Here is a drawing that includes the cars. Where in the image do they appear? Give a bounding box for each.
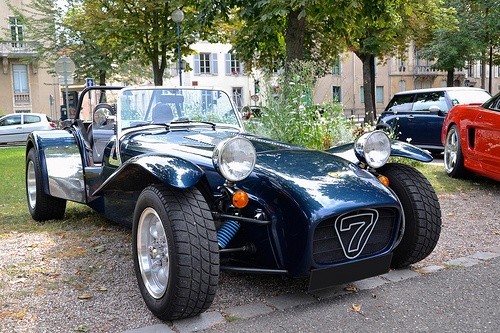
[442,91,500,183]
[376,85,493,157]
[0,113,55,145]
[23,84,441,323]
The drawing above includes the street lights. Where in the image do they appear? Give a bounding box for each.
[171,9,186,87]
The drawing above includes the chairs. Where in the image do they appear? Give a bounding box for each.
[152,102,174,122]
